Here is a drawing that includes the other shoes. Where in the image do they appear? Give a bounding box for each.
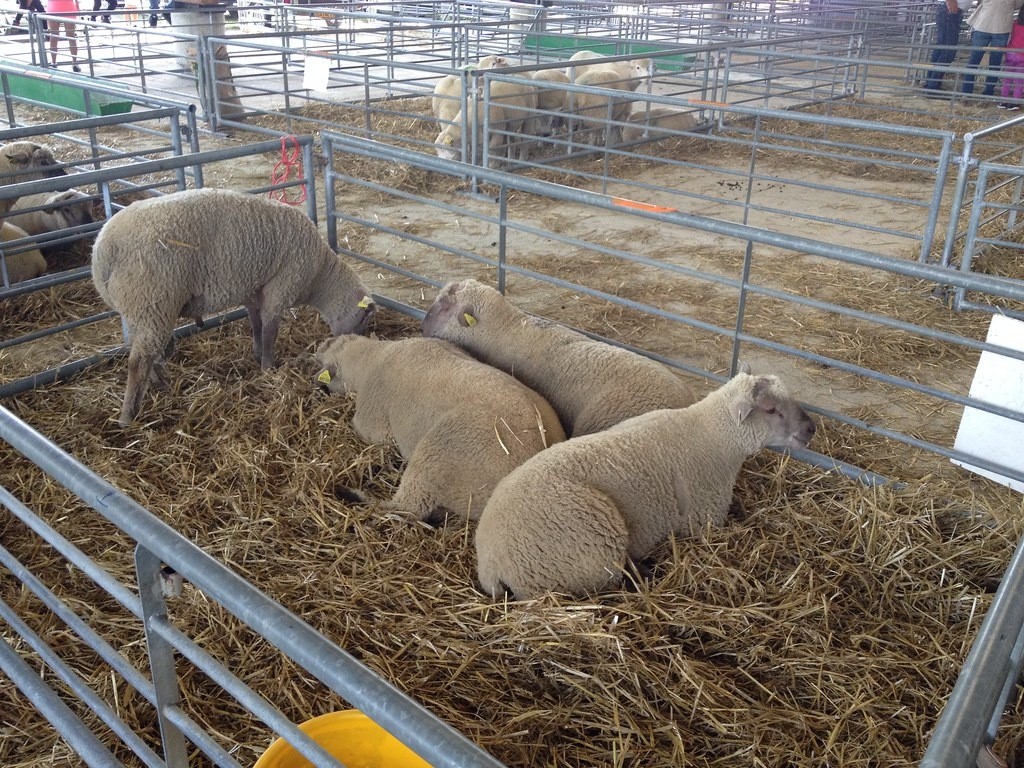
[101,16,110,24]
[1008,103,1019,111]
[51,63,57,68]
[163,13,171,24]
[148,15,158,27]
[997,102,1007,108]
[74,64,81,71]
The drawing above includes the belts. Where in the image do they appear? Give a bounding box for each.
[941,1,964,14]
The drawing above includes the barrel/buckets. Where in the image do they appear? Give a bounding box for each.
[253,709,434,768]
[171,13,225,68]
[510,8,547,31]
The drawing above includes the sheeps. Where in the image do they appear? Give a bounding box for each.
[310,334,568,524]
[0,220,47,287]
[90,187,378,430]
[473,361,815,602]
[0,141,71,233]
[5,190,104,235]
[419,279,697,440]
[432,50,698,167]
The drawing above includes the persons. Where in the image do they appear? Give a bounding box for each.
[961,0,1024,106]
[91,0,117,28]
[997,5,1024,110]
[149,0,174,26]
[923,0,974,100]
[47,0,81,71]
[12,0,50,41]
